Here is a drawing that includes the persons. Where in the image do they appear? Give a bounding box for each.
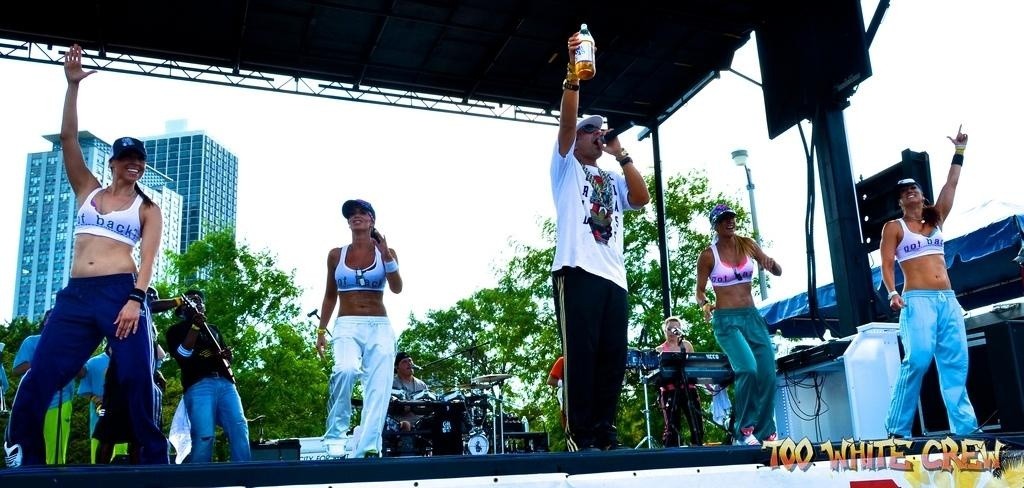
[3,42,170,470]
[879,123,986,437]
[547,27,650,453]
[545,355,566,431]
[384,352,428,459]
[76,320,170,467]
[692,203,783,448]
[11,308,89,465]
[314,198,403,459]
[652,316,707,448]
[153,290,255,464]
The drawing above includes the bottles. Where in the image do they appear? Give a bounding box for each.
[574,24,597,80]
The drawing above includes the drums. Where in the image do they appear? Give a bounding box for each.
[640,346,659,378]
[462,426,492,455]
[626,345,643,373]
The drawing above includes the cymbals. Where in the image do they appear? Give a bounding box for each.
[457,385,491,388]
[473,374,512,382]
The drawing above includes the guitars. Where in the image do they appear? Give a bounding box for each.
[180,293,236,384]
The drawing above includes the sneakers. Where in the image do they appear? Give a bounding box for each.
[885,423,904,438]
[365,449,379,457]
[768,434,776,441]
[731,426,761,446]
[608,444,634,450]
[583,447,601,452]
[326,444,345,458]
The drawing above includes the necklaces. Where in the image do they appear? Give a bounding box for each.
[903,214,925,224]
[99,185,139,216]
[718,240,742,280]
[578,160,614,213]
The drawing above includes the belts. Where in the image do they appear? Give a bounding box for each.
[204,371,223,377]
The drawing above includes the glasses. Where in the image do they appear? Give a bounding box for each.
[581,124,605,134]
[349,208,368,215]
[357,270,366,286]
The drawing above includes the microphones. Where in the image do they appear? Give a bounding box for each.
[598,120,635,142]
[666,325,685,337]
[409,364,425,373]
[306,308,320,317]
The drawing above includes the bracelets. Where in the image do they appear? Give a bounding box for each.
[315,328,326,333]
[702,300,710,311]
[954,145,965,155]
[951,153,964,166]
[614,148,628,161]
[565,62,580,82]
[128,288,145,302]
[619,157,632,166]
[191,323,201,331]
[382,259,398,273]
[887,291,898,300]
[560,78,579,91]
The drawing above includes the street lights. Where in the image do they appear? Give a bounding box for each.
[731,150,768,301]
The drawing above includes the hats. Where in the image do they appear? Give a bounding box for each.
[342,199,375,221]
[394,352,410,374]
[898,178,921,189]
[709,205,736,230]
[175,289,204,318]
[576,115,602,132]
[109,137,147,161]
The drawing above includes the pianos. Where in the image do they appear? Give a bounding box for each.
[388,395,471,415]
[656,351,736,383]
